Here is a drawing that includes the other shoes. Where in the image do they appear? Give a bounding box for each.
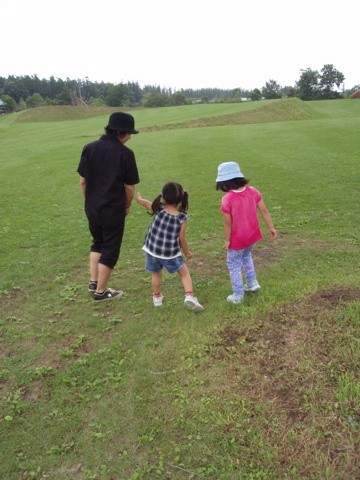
[225,293,243,305]
[152,294,162,307]
[184,296,204,313]
[245,284,260,292]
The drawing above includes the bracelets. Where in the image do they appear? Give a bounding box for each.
[125,203,131,208]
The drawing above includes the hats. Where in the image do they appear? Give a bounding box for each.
[216,162,244,182]
[106,112,139,134]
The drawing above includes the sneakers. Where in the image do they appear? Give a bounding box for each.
[93,289,124,302]
[89,280,97,291]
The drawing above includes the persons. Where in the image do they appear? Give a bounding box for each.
[216,161,277,304]
[77,112,139,301]
[135,182,204,313]
